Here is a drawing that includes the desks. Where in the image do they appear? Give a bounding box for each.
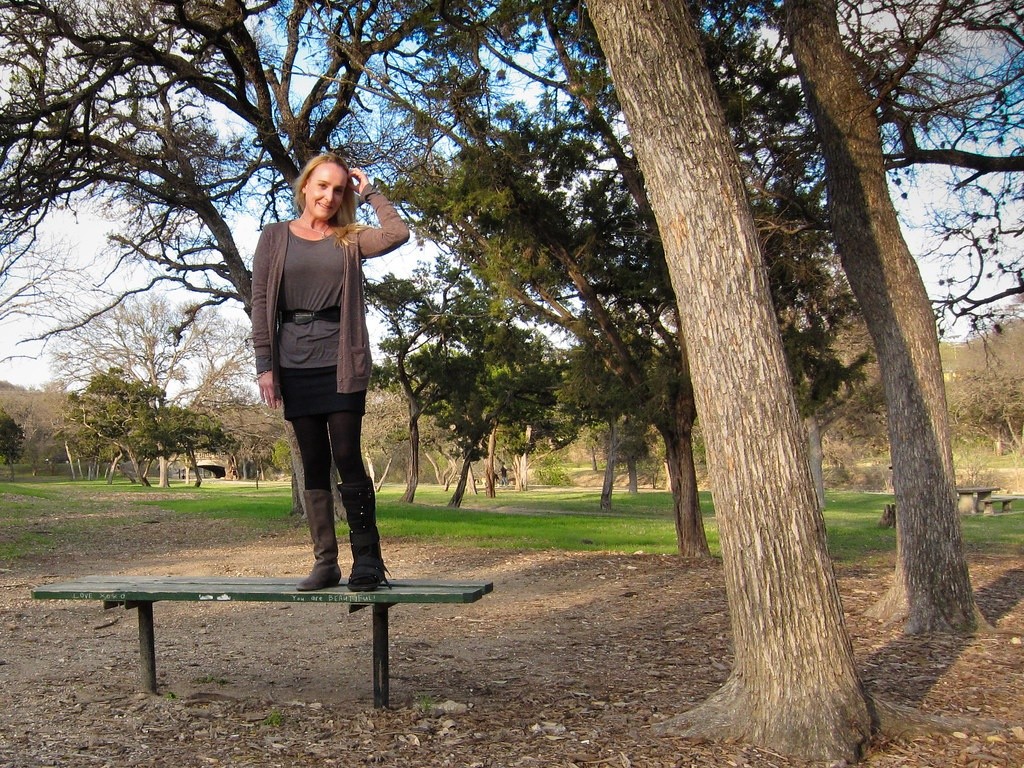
[957,488,1000,514]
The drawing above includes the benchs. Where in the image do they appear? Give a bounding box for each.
[981,497,1018,515]
[31,576,494,712]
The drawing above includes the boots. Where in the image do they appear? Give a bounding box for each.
[338,477,392,590]
[297,489,341,590]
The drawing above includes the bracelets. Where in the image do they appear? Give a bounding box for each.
[255,371,268,380]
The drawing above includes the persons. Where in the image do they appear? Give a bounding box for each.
[251,154,410,591]
[500,466,507,484]
